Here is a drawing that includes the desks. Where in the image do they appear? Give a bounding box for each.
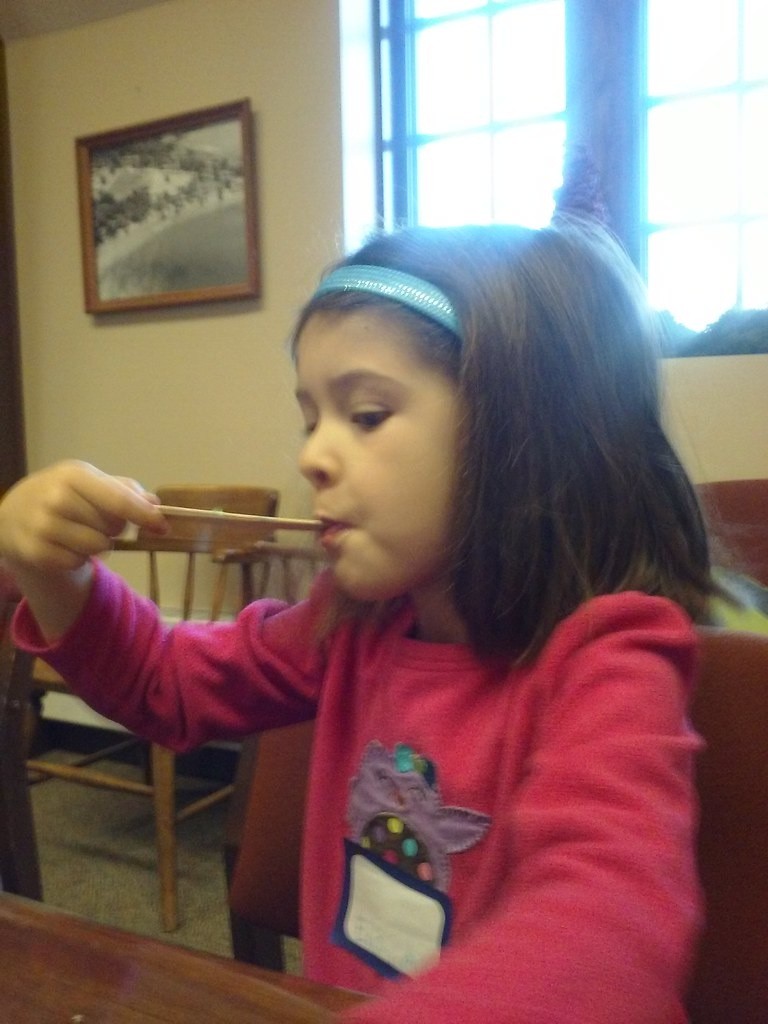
[0,894,373,1024]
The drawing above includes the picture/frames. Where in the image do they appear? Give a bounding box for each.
[74,97,261,315]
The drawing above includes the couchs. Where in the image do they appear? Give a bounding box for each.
[230,477,768,1024]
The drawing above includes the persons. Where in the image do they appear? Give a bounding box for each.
[0,221,715,1022]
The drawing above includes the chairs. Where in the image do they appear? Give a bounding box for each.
[9,486,282,937]
